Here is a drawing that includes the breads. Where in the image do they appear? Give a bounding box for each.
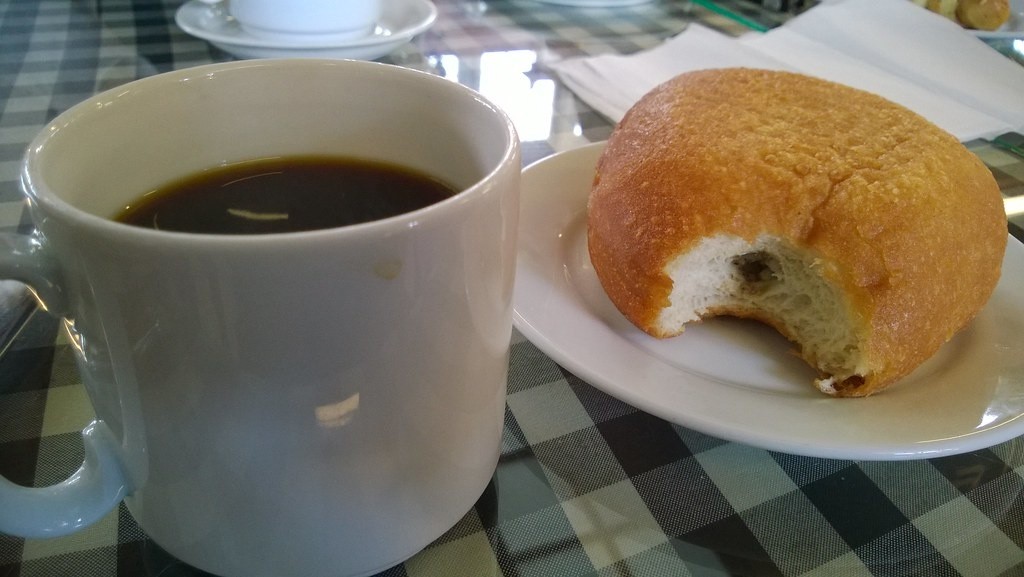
[587,67,1009,400]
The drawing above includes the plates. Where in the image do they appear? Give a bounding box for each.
[175,0,439,60]
[513,137,1023,466]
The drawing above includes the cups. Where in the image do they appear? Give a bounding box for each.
[223,0,382,42]
[4,56,525,573]
